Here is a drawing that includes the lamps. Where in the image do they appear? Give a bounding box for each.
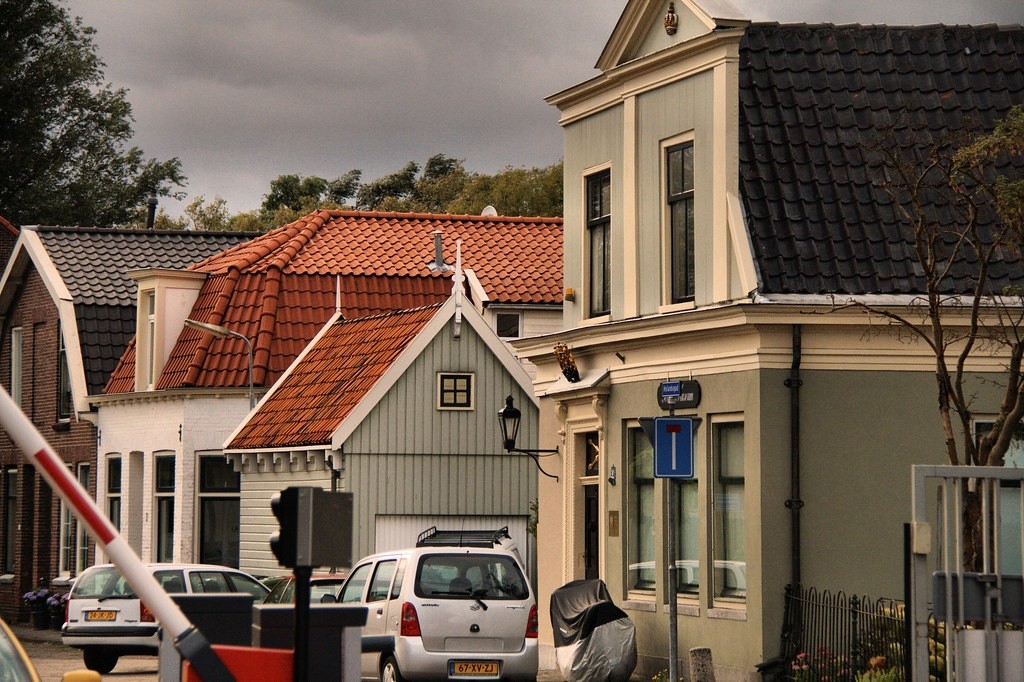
[498,393,559,482]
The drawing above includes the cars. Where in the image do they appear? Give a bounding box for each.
[0,617,102,681]
[259,572,398,605]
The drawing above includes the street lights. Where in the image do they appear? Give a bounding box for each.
[183,318,255,411]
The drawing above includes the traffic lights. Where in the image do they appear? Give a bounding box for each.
[268,484,302,570]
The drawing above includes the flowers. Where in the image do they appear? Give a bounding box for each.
[791,644,854,682]
[23,586,54,611]
[855,654,896,682]
[47,593,68,616]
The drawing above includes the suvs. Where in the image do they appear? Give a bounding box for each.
[58,563,279,677]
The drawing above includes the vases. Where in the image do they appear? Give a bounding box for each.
[49,616,64,632]
[31,610,49,630]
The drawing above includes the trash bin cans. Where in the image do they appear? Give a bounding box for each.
[157,592,258,682]
[252,602,369,682]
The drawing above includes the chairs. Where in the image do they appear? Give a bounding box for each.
[447,579,472,596]
[203,580,220,591]
[164,581,182,593]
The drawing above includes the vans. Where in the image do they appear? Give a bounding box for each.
[416,526,526,601]
[320,546,540,682]
